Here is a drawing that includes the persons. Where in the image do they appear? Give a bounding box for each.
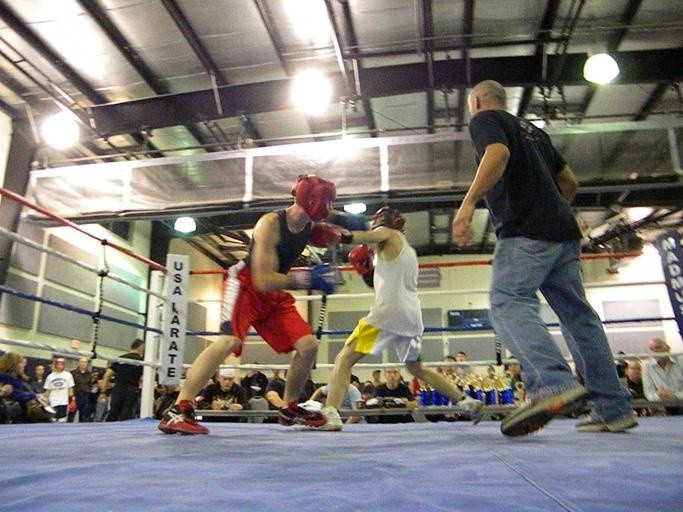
[308,351,528,424]
[0,338,143,425]
[452,79,639,435]
[180,364,329,424]
[615,339,683,417]
[159,176,375,435]
[295,207,484,430]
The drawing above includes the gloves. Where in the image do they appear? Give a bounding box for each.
[348,244,375,289]
[365,397,384,409]
[384,397,406,409]
[309,223,353,248]
[290,263,336,294]
[67,396,76,413]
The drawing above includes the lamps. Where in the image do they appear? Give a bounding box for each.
[174,217,197,233]
[344,203,367,215]
[583,35,620,85]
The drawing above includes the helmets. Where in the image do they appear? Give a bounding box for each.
[291,173,336,221]
[371,208,407,246]
[55,357,66,371]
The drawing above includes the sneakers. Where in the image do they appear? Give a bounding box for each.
[575,407,639,432]
[158,400,209,435]
[278,402,327,427]
[296,406,343,431]
[457,393,485,425]
[500,381,588,436]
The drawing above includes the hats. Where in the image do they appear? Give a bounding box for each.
[219,368,235,378]
